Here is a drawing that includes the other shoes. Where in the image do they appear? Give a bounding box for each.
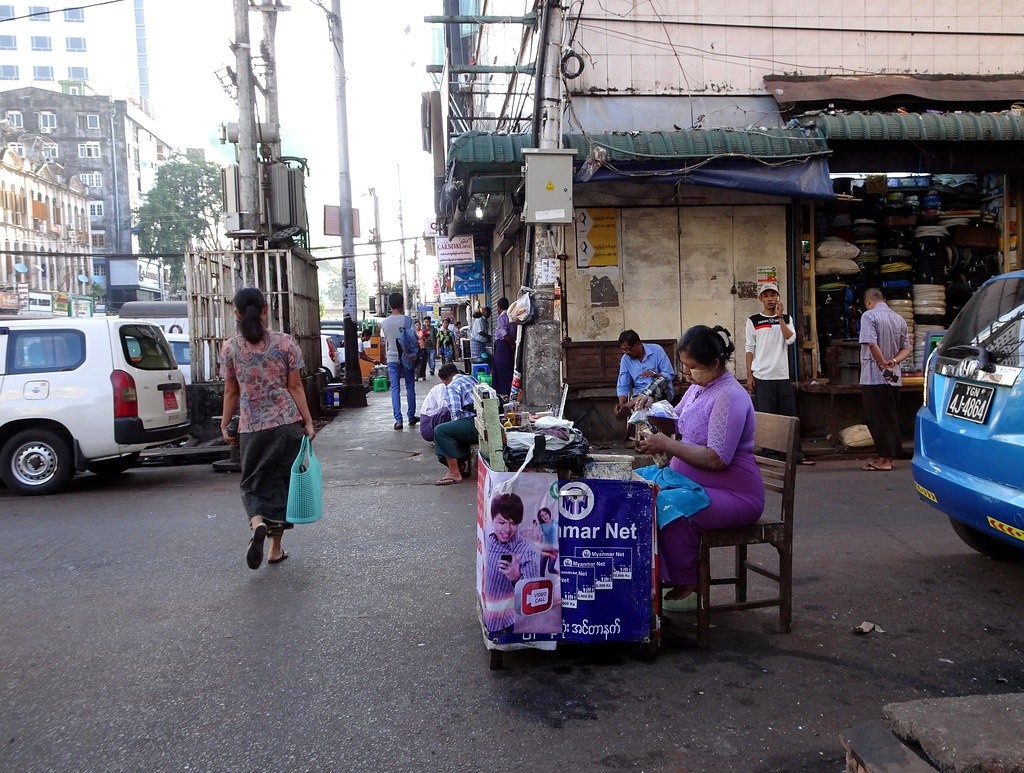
[423,377,426,380]
[430,370,434,375]
[415,379,418,381]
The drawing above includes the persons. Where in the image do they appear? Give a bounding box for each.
[493,297,517,401]
[220,288,315,570]
[358,329,380,364]
[434,364,480,485]
[420,370,466,448]
[639,324,765,601]
[530,507,559,577]
[422,316,438,375]
[486,491,540,634]
[436,317,459,365]
[744,283,816,465]
[470,307,492,358]
[614,330,675,444]
[859,288,911,470]
[453,321,466,361]
[381,292,420,430]
[415,321,428,381]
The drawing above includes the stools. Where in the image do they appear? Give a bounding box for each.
[374,377,388,392]
[472,363,489,378]
[477,374,489,382]
[326,383,343,405]
[481,377,492,386]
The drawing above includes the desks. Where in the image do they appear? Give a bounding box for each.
[475,450,658,670]
[792,379,929,447]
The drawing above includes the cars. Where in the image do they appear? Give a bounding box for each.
[320,323,370,381]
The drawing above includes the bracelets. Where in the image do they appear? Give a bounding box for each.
[893,359,897,366]
[512,574,524,588]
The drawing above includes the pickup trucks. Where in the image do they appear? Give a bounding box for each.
[0,318,193,497]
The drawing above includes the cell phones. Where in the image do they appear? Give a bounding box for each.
[882,369,899,383]
[501,555,512,570]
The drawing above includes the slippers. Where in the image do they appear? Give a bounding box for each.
[409,416,420,426]
[394,423,403,429]
[267,548,287,563]
[863,463,891,471]
[797,458,816,465]
[246,523,266,570]
[436,478,463,486]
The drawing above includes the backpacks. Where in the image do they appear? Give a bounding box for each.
[395,316,420,369]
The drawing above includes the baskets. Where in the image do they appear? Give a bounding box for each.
[286,435,323,525]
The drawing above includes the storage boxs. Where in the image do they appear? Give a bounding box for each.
[582,454,635,480]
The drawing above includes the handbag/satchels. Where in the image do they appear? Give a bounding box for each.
[506,292,530,324]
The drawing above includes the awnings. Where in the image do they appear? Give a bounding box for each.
[443,112,1024,202]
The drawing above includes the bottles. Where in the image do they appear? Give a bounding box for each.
[502,401,532,427]
[641,426,670,469]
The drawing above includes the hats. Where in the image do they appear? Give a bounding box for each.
[760,284,778,296]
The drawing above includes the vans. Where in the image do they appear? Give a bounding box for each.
[120,300,225,387]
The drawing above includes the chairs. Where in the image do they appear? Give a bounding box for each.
[691,409,800,640]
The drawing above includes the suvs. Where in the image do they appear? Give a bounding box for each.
[911,268,1024,560]
